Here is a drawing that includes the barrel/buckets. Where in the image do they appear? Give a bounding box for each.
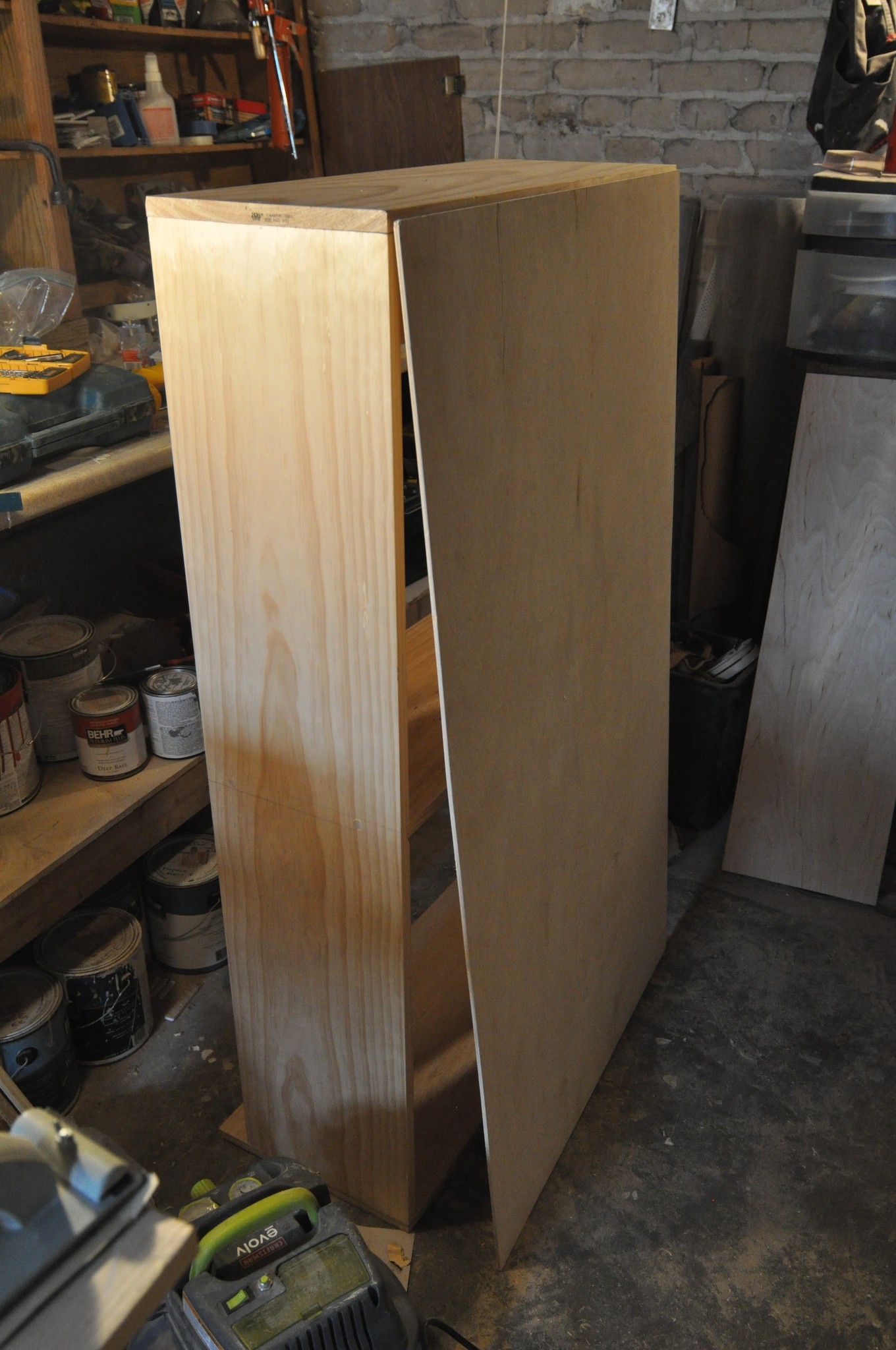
[0,613,205,818]
[0,830,228,1118]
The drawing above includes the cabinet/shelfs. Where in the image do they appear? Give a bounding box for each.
[0,0,323,354]
[0,431,212,966]
[143,159,679,1225]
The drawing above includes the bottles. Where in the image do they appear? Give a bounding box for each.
[80,62,119,105]
[136,52,181,146]
